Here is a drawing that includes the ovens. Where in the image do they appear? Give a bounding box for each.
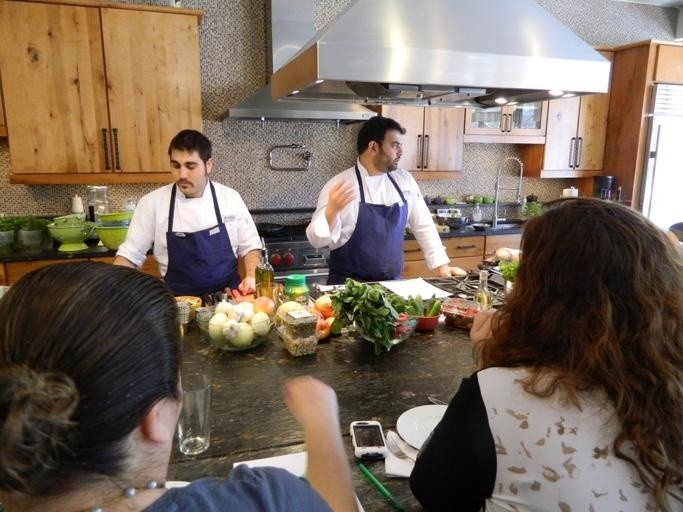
[273,268,329,286]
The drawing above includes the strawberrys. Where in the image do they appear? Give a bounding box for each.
[440,296,477,331]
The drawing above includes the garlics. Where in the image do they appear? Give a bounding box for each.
[208,302,269,348]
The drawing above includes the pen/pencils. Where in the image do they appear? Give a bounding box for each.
[361,465,405,512]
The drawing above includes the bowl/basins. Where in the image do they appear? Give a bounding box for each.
[423,194,494,206]
[172,296,202,318]
[437,216,466,229]
[45,211,134,251]
[472,223,489,230]
[196,306,276,351]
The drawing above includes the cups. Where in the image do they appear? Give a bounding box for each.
[175,373,211,456]
[256,280,278,304]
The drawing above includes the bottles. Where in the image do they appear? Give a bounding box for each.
[473,271,491,307]
[70,193,83,213]
[521,195,526,211]
[283,275,308,307]
[175,303,191,324]
[254,247,274,298]
[472,204,482,222]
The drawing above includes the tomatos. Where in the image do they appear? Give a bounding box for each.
[253,295,274,314]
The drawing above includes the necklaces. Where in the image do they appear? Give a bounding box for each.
[88,480,165,512]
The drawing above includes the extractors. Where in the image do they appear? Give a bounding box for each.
[267,0,609,107]
[217,0,377,126]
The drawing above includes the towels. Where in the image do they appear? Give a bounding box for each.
[385,430,419,479]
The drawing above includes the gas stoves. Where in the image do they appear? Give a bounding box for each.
[262,223,330,268]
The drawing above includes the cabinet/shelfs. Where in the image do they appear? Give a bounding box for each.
[1,0,204,183]
[5,256,163,288]
[485,234,519,258]
[465,103,546,145]
[382,104,464,180]
[401,237,485,281]
[519,45,615,180]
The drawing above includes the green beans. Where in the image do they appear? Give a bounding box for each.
[406,294,441,317]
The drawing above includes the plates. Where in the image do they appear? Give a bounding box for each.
[395,405,451,450]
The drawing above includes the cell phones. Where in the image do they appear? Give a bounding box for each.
[349,420,387,459]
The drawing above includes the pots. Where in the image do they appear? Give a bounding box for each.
[258,222,282,233]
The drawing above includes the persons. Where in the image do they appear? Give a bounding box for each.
[2,259,354,512]
[408,198,683,512]
[107,126,268,294]
[304,114,466,285]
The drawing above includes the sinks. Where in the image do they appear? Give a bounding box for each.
[466,218,525,231]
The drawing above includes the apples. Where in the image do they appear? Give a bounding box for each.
[315,293,337,339]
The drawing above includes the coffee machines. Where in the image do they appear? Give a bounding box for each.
[593,175,615,201]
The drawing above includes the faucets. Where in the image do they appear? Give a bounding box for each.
[491,156,524,230]
